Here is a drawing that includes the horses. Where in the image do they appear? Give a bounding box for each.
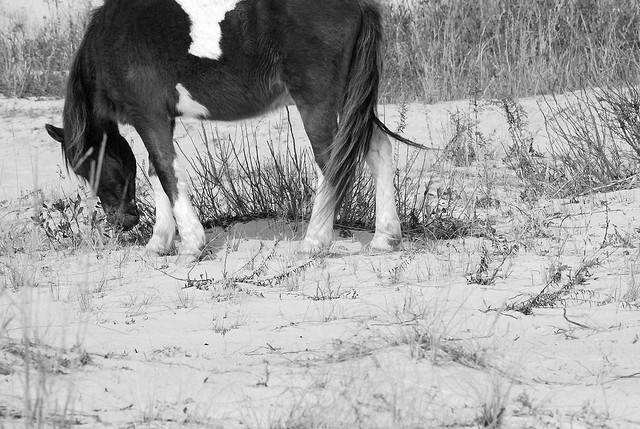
[47,0,441,264]
